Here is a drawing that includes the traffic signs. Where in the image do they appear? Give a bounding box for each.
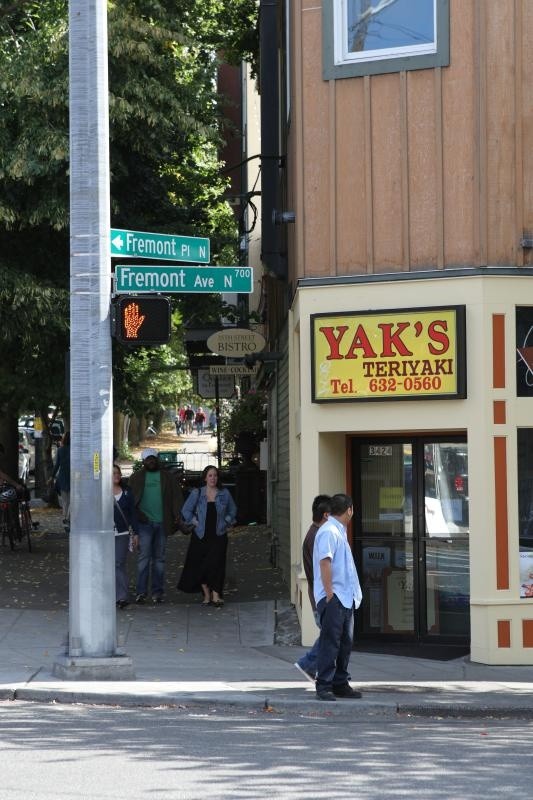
[109,228,210,265]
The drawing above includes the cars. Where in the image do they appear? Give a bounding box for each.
[400,440,533,611]
[18,413,65,477]
[19,429,32,486]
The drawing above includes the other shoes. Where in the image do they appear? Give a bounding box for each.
[200,599,212,607]
[152,596,164,604]
[332,680,362,698]
[135,592,146,604]
[115,598,130,608]
[211,596,225,607]
[295,657,317,683]
[62,518,70,533]
[316,686,336,701]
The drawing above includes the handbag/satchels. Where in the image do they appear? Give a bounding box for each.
[180,488,201,535]
[128,532,137,553]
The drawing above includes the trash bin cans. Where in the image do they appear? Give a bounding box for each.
[158,451,178,467]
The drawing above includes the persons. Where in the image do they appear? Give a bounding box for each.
[180,465,237,606]
[293,493,363,703]
[128,447,182,605]
[46,431,71,532]
[175,404,218,437]
[0,470,25,491]
[113,464,140,609]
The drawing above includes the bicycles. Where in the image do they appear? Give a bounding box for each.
[0,480,40,554]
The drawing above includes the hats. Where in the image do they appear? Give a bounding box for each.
[141,448,157,460]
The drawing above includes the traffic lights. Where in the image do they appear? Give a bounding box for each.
[116,295,172,345]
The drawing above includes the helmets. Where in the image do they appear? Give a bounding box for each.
[0,488,16,502]
